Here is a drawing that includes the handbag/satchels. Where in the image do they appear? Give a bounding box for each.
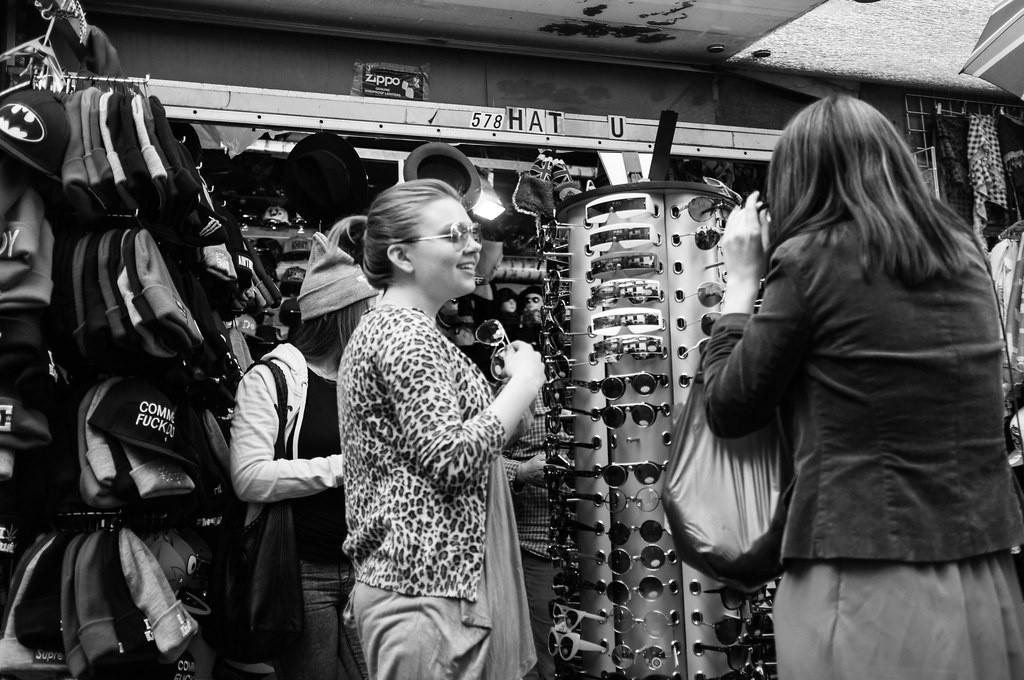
[201,360,306,663]
[660,348,795,590]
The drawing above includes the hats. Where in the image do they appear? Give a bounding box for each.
[512,151,582,215]
[263,132,378,341]
[403,142,481,209]
[0,88,282,680]
[495,286,545,308]
[1007,406,1024,467]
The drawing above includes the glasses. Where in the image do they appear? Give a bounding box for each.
[406,223,483,252]
[523,176,777,680]
[476,319,517,381]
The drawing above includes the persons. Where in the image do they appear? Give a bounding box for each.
[501,387,565,680]
[702,94,1023,679]
[230,231,380,680]
[325,179,544,680]
